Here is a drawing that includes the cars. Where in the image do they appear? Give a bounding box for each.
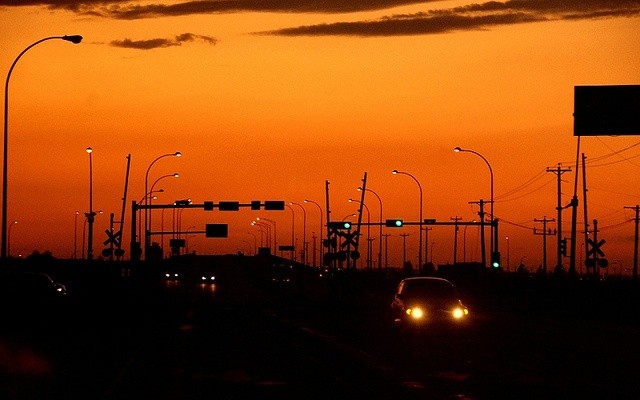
[319,267,334,279]
[33,273,66,298]
[165,267,180,278]
[390,276,472,338]
[200,271,215,282]
[272,266,298,284]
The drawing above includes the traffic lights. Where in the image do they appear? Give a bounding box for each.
[218,201,238,212]
[386,219,403,228]
[264,201,286,209]
[328,222,351,229]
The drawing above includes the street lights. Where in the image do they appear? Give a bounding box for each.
[253,221,271,255]
[464,220,477,265]
[505,235,511,271]
[290,202,305,265]
[74,211,79,258]
[392,170,422,273]
[454,146,493,267]
[257,217,276,256]
[340,214,356,268]
[348,199,370,269]
[8,220,18,256]
[357,187,382,269]
[285,205,294,261]
[249,232,257,256]
[304,200,322,268]
[84,147,103,259]
[251,222,263,247]
[138,152,196,257]
[243,240,251,255]
